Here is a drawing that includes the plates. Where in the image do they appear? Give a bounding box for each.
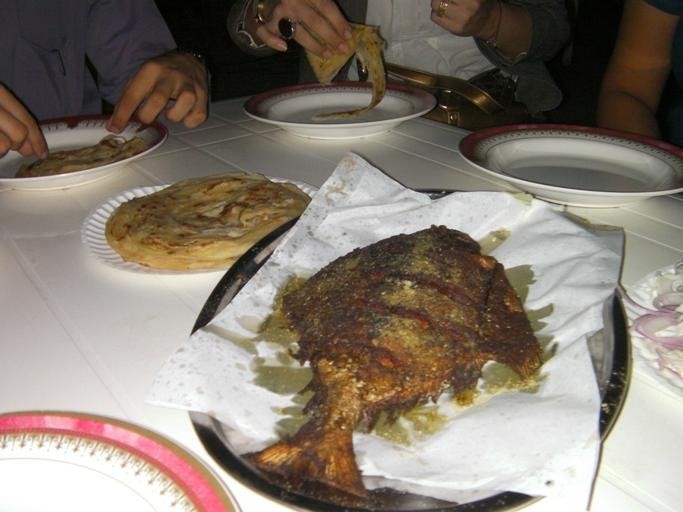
[0,113,170,189]
[241,83,438,142]
[457,123,683,209]
[184,189,632,512]
[0,413,240,512]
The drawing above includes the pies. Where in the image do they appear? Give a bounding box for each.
[107,171,311,270]
[14,135,146,180]
[302,19,388,121]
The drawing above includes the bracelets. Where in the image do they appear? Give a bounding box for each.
[482,1,504,49]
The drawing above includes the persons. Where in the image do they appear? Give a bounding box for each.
[0,1,214,162]
[226,2,575,111]
[596,0,683,150]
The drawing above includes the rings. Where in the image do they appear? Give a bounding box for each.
[275,14,297,43]
[435,1,450,20]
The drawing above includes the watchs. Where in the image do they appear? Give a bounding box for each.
[250,0,267,26]
[164,46,211,73]
